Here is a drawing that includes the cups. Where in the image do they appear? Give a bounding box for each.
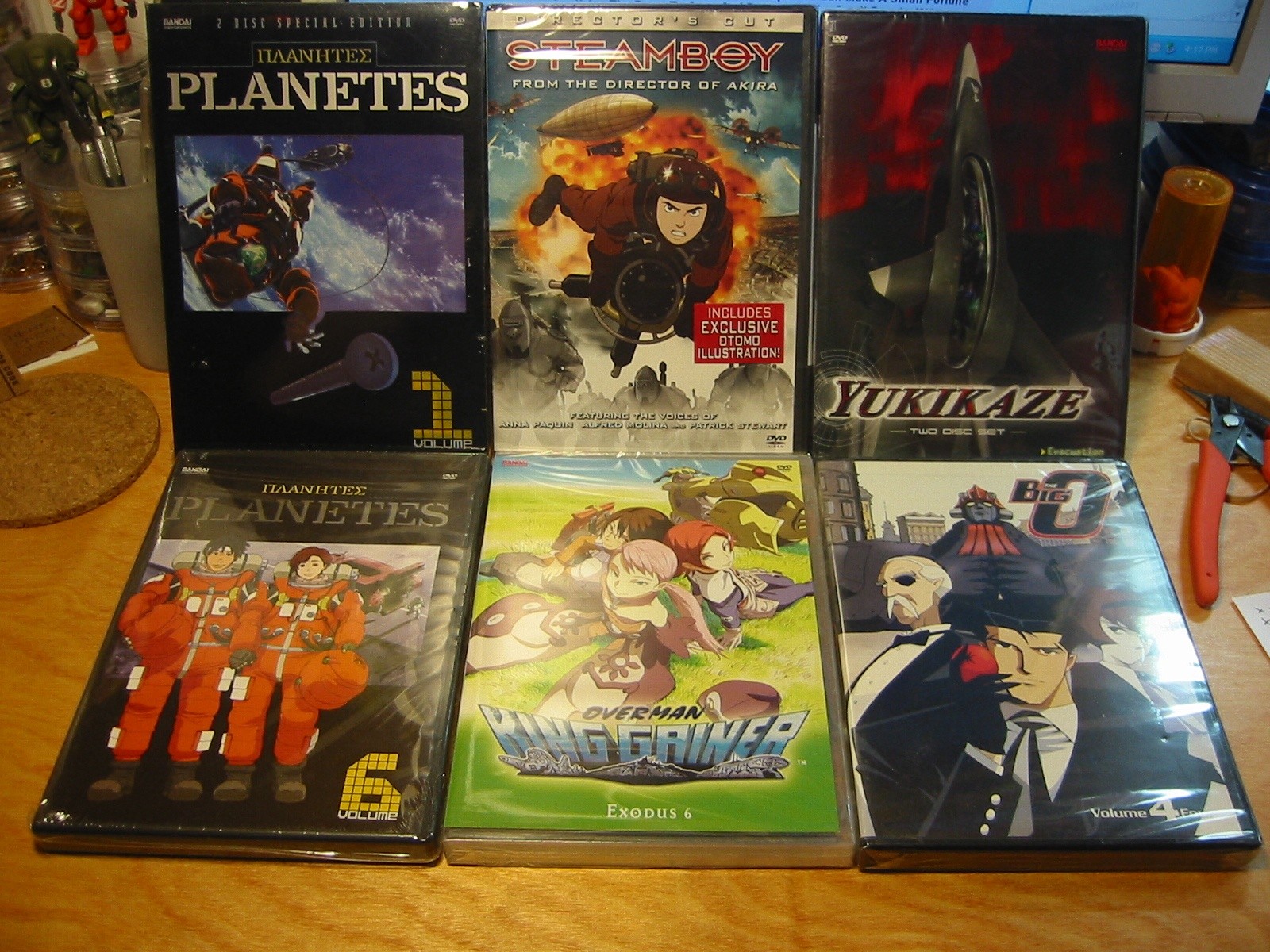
[75,138,168,372]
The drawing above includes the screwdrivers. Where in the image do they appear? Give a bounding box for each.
[1181,386,1270,440]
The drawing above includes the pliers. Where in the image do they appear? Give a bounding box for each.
[1188,395,1270,606]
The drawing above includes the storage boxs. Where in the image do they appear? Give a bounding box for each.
[34,444,491,866]
[815,11,1148,455]
[444,452,856,872]
[488,3,817,449]
[143,1,486,449]
[824,459,1262,875]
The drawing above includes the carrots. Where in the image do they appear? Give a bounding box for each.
[1133,264,1202,323]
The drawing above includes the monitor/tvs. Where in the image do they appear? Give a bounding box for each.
[295,0,1270,126]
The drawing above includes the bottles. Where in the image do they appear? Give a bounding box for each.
[74,30,150,117]
[0,148,57,294]
[22,118,142,332]
[1132,166,1234,357]
